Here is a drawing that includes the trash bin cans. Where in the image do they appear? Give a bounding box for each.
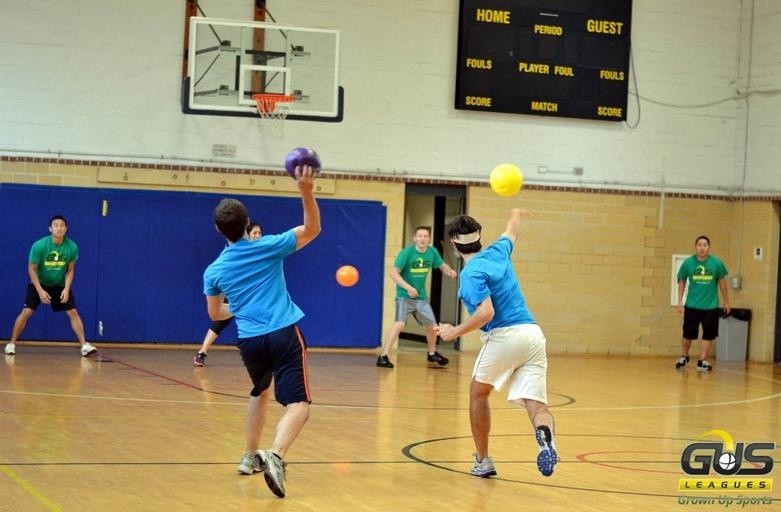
[716,306,750,361]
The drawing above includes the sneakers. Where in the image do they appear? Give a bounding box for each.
[674,354,690,369]
[3,343,18,356]
[253,449,288,498]
[237,451,263,475]
[376,354,395,368]
[78,340,99,357]
[468,452,499,478]
[193,350,206,367]
[427,350,449,366]
[697,360,713,372]
[534,424,558,476]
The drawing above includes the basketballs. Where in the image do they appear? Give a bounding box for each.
[491,164,522,196]
[337,265,359,287]
[286,148,322,179]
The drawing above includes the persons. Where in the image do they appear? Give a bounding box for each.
[675,236,731,372]
[433,207,561,478]
[193,220,263,367]
[203,165,322,498]
[377,227,457,368]
[5,214,98,357]
